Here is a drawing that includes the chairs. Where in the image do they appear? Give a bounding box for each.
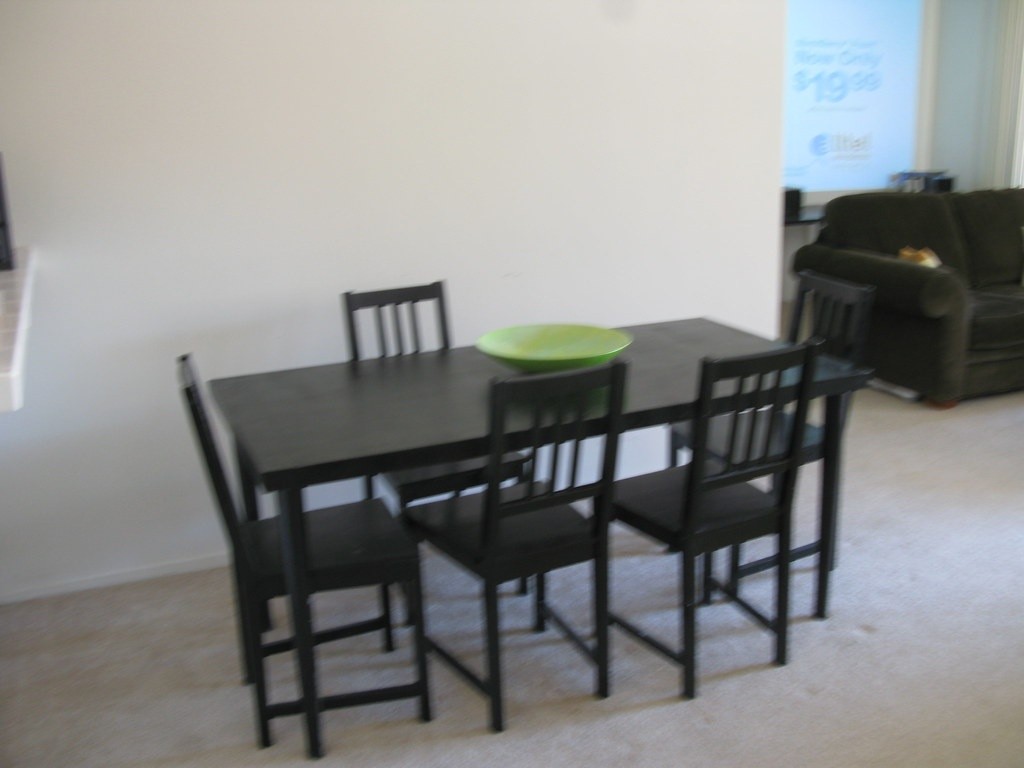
[665,277,884,604]
[598,335,821,700]
[344,281,539,623]
[405,359,628,731]
[174,354,431,751]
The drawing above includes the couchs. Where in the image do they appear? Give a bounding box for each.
[794,188,1024,410]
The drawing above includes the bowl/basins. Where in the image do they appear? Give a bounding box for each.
[476,324,633,373]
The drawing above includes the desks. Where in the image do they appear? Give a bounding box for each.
[210,318,873,760]
